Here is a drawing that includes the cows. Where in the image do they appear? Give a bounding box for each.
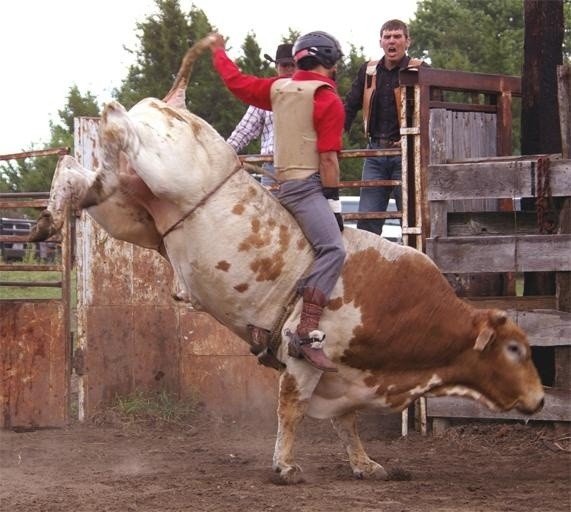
[25,33,547,485]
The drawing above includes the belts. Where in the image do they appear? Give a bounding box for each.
[375,138,400,147]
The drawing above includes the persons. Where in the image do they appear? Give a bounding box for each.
[343,20,443,235]
[226,44,295,199]
[208,31,346,372]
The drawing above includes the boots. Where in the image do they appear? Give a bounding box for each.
[288,285,339,372]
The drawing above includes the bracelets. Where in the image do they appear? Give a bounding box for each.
[326,198,343,213]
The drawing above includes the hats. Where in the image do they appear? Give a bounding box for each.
[264,43,295,64]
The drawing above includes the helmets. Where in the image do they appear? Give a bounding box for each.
[291,32,341,68]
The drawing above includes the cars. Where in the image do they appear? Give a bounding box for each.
[338,195,404,244]
